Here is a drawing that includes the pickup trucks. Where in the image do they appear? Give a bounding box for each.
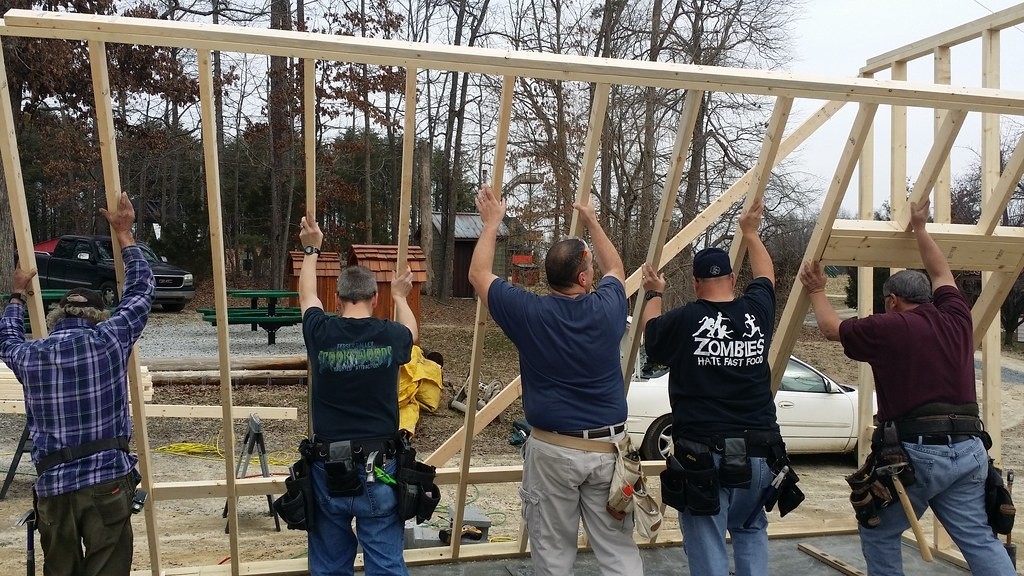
[15,234,195,311]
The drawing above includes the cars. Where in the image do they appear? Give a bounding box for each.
[625,332,882,461]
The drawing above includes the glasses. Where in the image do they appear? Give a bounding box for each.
[577,238,588,275]
[881,294,897,305]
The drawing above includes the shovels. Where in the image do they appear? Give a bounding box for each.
[877,462,934,562]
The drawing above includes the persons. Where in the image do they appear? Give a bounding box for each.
[0,192,158,576]
[298,210,417,576]
[468,184,643,576]
[639,200,805,576]
[800,194,1018,576]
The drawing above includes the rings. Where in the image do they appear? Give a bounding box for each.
[642,275,646,279]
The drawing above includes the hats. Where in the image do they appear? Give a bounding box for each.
[59,288,104,312]
[693,248,732,278]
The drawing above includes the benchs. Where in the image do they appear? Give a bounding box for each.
[23,307,115,330]
[195,307,339,324]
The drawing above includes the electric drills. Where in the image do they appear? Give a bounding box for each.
[438,515,483,545]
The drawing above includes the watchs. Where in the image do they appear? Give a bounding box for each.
[304,246,321,256]
[9,293,27,304]
[645,290,662,300]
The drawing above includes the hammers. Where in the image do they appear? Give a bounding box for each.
[15,509,35,576]
[365,451,397,487]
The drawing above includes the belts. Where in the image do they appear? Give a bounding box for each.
[900,433,975,446]
[547,424,624,439]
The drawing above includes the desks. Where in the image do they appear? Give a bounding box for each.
[0,289,101,331]
[229,290,287,334]
[231,292,299,346]
